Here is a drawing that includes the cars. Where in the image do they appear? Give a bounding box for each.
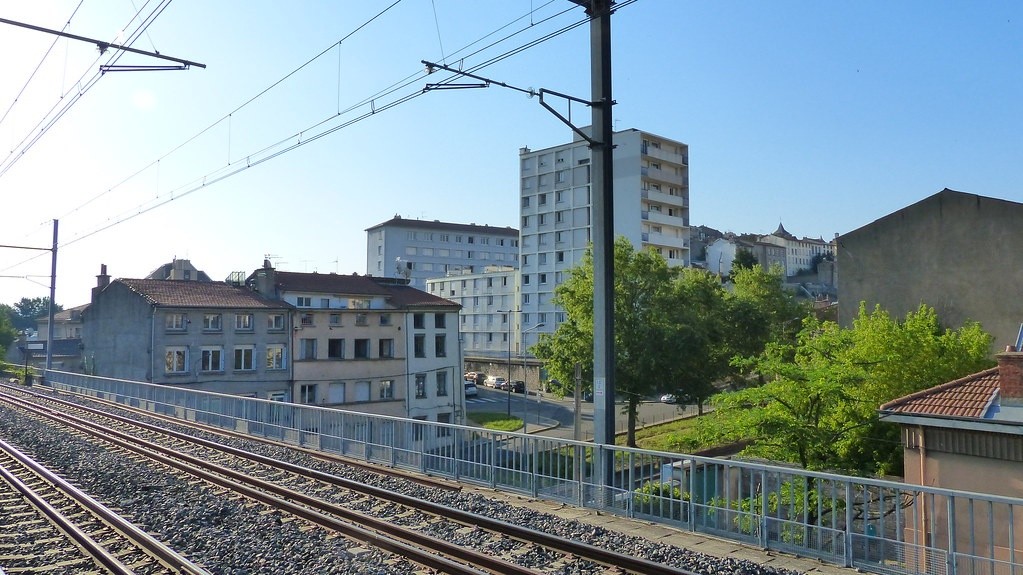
[483,376,506,388]
[500,379,524,392]
[661,392,679,405]
[464,380,478,396]
[464,372,487,384]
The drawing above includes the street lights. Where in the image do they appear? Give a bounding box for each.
[521,324,545,434]
[496,310,525,417]
